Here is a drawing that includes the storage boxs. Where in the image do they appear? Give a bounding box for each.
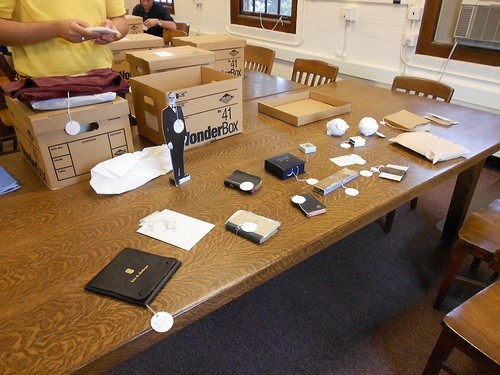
[126,45,216,76]
[129,65,243,152]
[172,33,246,78]
[110,33,164,81]
[258,90,352,127]
[125,14,143,34]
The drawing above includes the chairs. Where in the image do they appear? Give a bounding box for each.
[291,58,339,87]
[176,22,190,36]
[244,44,275,74]
[384,76,455,234]
[163,29,187,48]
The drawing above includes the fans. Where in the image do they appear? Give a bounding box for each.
[4,96,135,191]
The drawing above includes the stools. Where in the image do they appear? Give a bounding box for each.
[433,207,500,310]
[421,277,500,375]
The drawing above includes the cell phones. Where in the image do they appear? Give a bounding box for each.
[86,27,118,35]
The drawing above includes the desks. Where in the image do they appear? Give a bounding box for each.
[242,67,309,102]
[0,80,500,375]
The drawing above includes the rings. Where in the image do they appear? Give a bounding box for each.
[82,37,84,41]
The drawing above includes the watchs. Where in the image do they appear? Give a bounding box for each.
[158,20,162,26]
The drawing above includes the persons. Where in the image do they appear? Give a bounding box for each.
[162,91,189,183]
[132,0,176,37]
[0,0,130,86]
